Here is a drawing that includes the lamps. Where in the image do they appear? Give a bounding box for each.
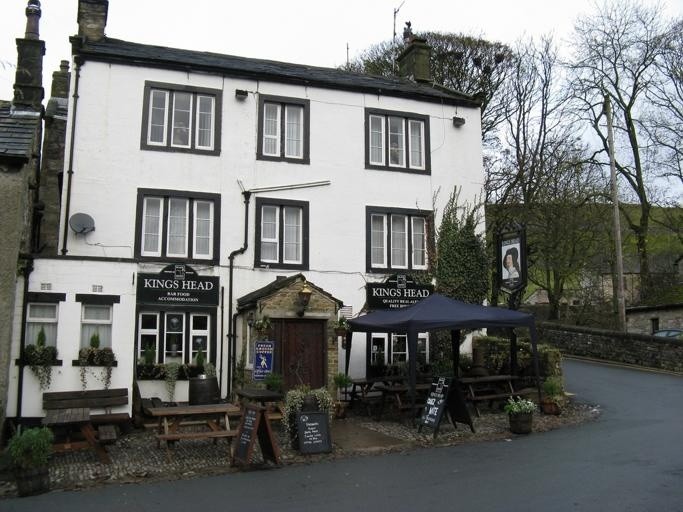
[296,280,312,307]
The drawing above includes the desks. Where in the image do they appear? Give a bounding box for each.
[349,373,519,426]
[146,403,241,461]
[235,389,284,403]
[39,409,112,464]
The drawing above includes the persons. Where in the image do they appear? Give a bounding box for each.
[502,247,519,279]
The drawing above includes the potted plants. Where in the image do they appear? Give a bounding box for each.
[328,317,353,336]
[3,424,59,499]
[538,373,569,416]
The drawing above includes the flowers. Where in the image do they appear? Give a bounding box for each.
[504,395,538,414]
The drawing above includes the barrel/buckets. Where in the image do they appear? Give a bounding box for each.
[188,374,220,404]
[11,461,50,499]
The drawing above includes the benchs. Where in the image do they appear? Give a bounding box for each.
[94,424,117,464]
[338,387,532,427]
[42,388,132,440]
[141,419,239,461]
